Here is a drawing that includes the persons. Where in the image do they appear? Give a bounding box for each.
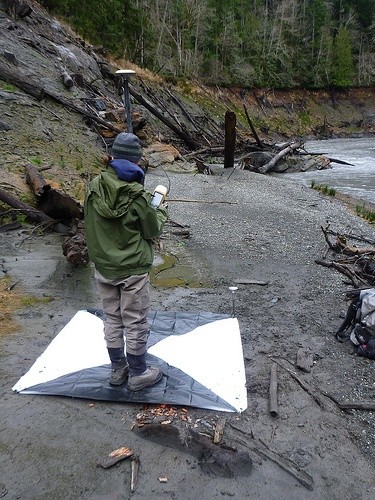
[81,129,169,392]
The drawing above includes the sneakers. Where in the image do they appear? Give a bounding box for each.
[127,367,162,391]
[109,364,129,386]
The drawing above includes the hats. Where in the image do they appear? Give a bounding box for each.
[112,133,143,162]
